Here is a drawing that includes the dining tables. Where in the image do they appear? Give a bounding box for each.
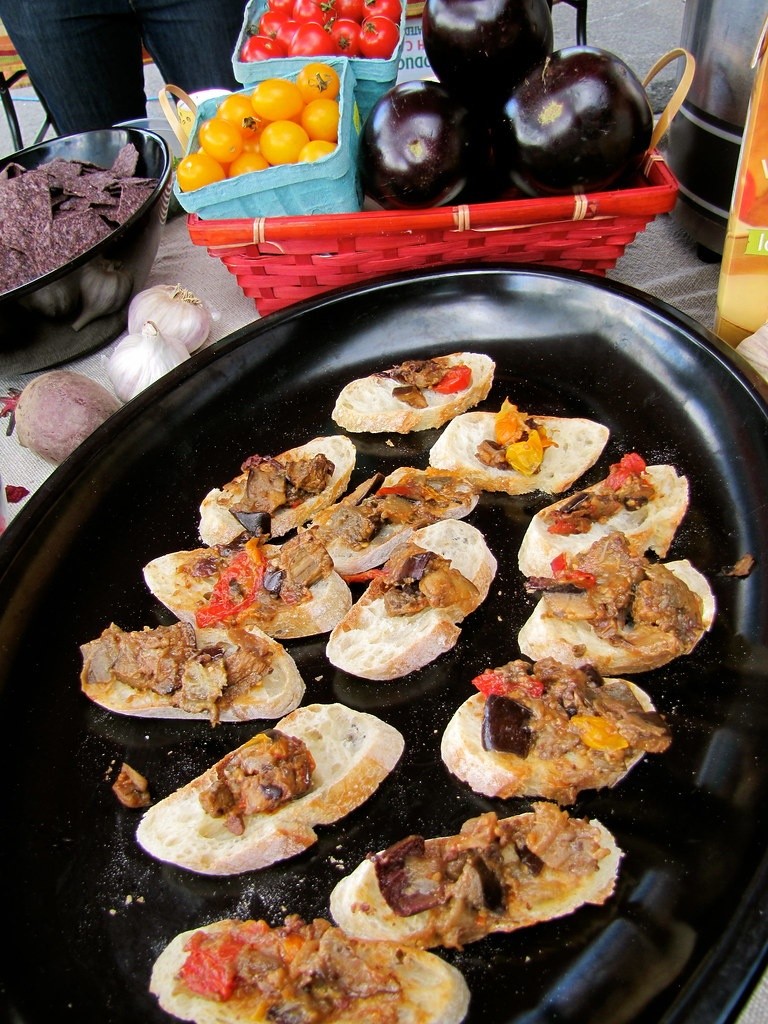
[0,85,767,1024]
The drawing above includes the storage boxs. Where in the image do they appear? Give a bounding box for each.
[157,44,696,325]
[228,19,406,124]
[171,55,365,221]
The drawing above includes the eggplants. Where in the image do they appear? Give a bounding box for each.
[420,0,556,111]
[498,43,655,195]
[359,80,493,211]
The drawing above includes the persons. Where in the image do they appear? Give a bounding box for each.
[0,0,249,140]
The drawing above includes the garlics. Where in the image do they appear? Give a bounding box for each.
[107,321,190,403]
[128,283,212,354]
[71,259,133,332]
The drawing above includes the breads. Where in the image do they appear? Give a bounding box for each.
[82,348,721,1024]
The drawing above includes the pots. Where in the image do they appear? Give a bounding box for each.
[665,0,767,264]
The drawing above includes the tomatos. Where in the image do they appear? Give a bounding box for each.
[176,0,403,192]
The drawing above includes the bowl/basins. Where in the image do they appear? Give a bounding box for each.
[0,126,174,378]
[113,119,187,225]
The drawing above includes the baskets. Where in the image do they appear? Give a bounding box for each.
[186,145,679,316]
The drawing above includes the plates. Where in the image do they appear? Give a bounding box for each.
[1,256,768,1024]
